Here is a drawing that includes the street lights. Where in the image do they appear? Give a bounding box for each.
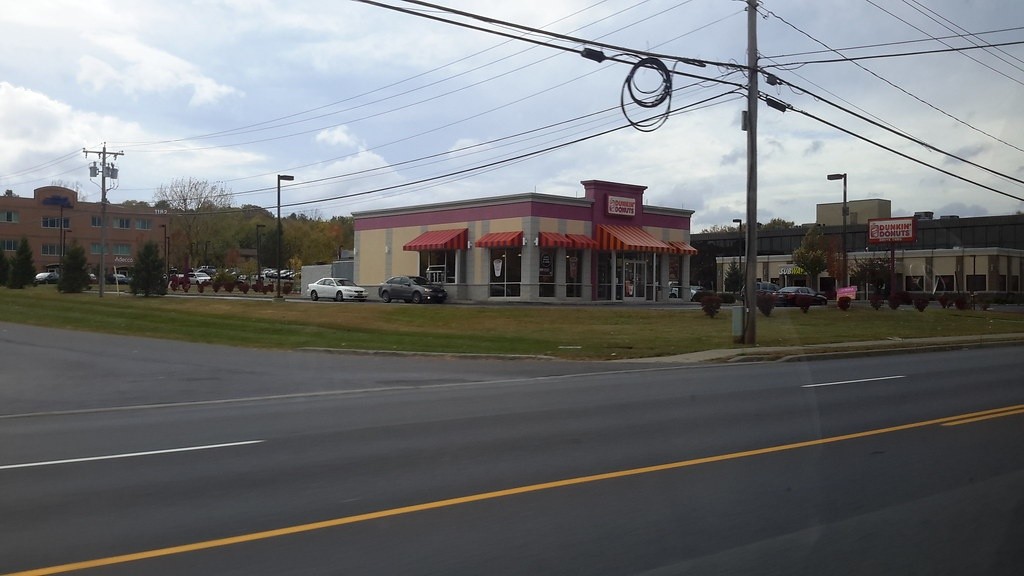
[159,224,170,285]
[827,172,848,289]
[733,218,742,296]
[63,230,72,256]
[89,161,119,299]
[272,174,294,302]
[205,241,211,265]
[58,205,75,290]
[256,223,266,293]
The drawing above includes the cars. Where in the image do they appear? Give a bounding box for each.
[773,286,828,307]
[89,274,97,282]
[306,278,369,302]
[186,273,212,286]
[377,275,448,303]
[108,273,128,285]
[741,281,781,303]
[34,272,58,284]
[171,266,302,280]
[669,281,696,300]
